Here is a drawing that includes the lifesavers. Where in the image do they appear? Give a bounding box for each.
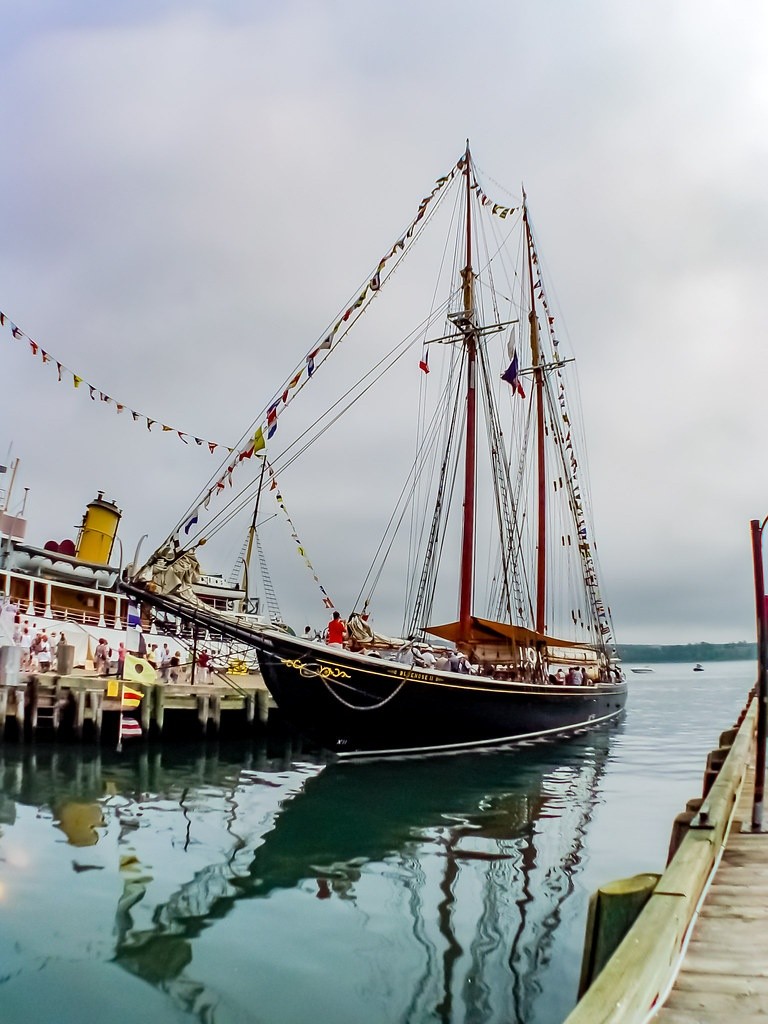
[527,648,537,664]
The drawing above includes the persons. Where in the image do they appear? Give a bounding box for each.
[14,611,626,686]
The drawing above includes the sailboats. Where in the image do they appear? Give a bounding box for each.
[113,134,629,768]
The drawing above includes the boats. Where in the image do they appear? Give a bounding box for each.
[631,668,655,673]
[693,664,704,671]
[1,438,265,672]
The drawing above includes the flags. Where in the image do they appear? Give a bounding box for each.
[121,687,145,709]
[122,716,142,738]
[500,325,526,400]
[184,512,198,535]
[419,346,430,374]
[128,601,143,628]
[266,408,277,440]
[253,426,266,454]
